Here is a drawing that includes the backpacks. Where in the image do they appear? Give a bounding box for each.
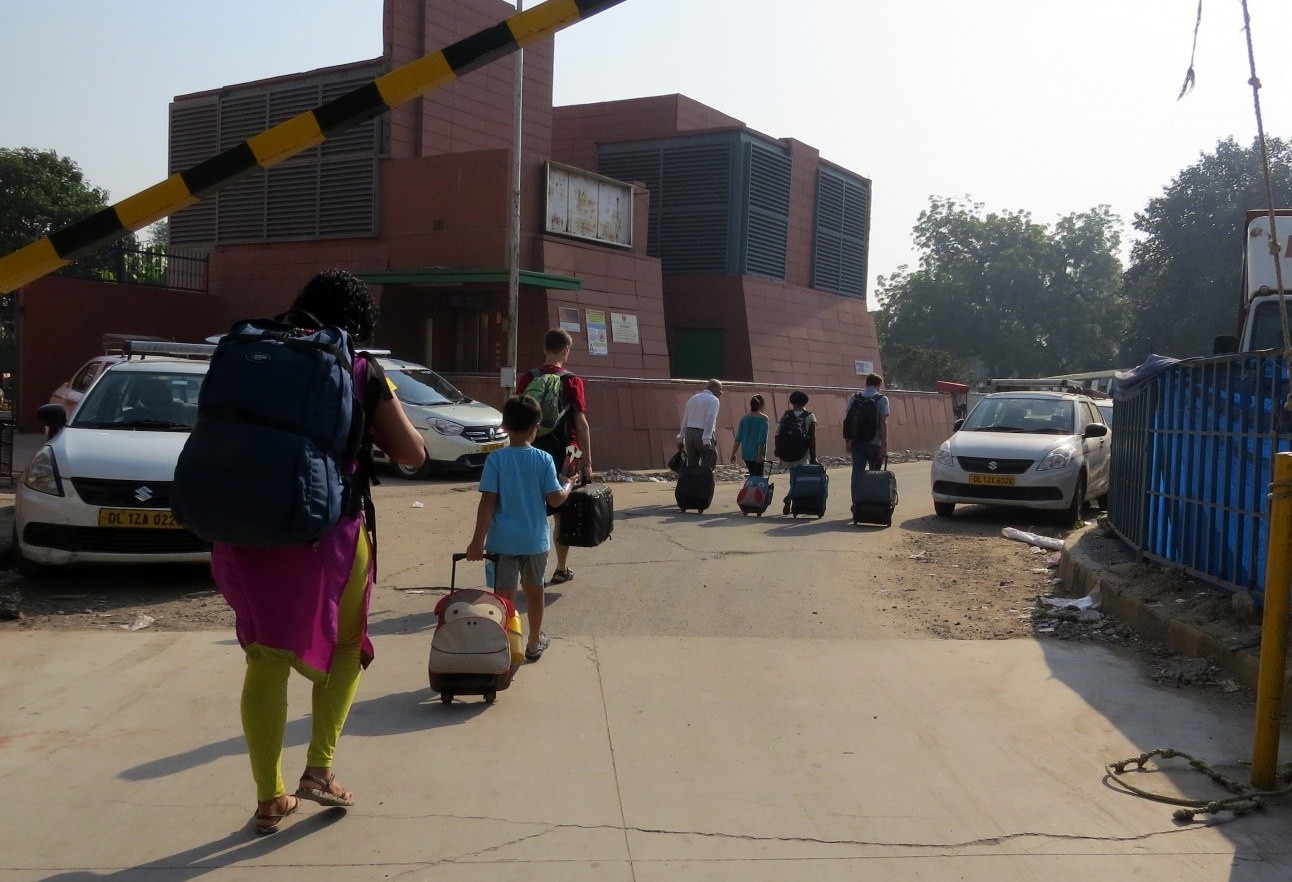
[169,311,352,544]
[779,410,812,462]
[522,369,570,436]
[842,393,882,441]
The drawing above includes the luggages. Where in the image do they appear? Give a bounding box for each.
[851,454,899,527]
[428,553,526,704]
[736,459,774,517]
[792,461,829,520]
[674,452,715,514]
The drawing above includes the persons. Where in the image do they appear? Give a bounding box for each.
[676,379,723,469]
[774,391,817,515]
[467,395,579,659]
[730,394,769,477]
[210,268,425,835]
[515,328,593,584]
[845,373,890,518]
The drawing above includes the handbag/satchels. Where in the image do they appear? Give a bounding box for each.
[668,450,688,475]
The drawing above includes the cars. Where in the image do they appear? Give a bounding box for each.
[360,358,509,480]
[1091,398,1113,430]
[930,390,1113,524]
[13,360,213,575]
[45,353,191,436]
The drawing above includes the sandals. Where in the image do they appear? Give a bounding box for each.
[255,794,301,835]
[296,771,355,806]
[525,630,549,660]
[550,566,575,585]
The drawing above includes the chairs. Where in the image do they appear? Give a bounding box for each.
[124,377,177,425]
[1047,415,1066,431]
[1004,409,1025,429]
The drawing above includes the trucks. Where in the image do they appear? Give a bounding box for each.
[1212,209,1292,396]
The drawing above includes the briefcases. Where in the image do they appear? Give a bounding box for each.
[554,477,614,547]
